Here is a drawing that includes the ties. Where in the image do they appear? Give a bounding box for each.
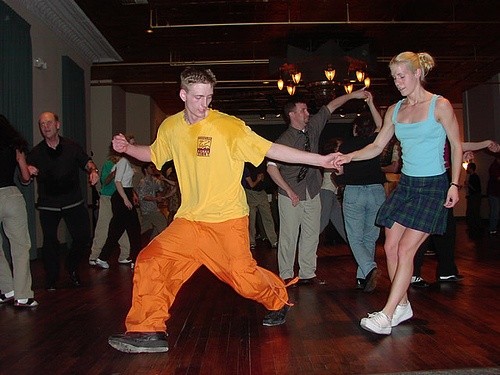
[296,130,311,184]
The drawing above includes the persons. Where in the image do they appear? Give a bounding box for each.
[337,90,387,290]
[488,143,500,153]
[466,163,481,232]
[267,86,370,288]
[134,156,167,233]
[106,64,345,352]
[88,140,132,265]
[332,52,463,335]
[156,166,182,225]
[410,135,500,287]
[96,136,140,268]
[26,112,99,290]
[241,161,279,251]
[0,114,42,308]
[487,156,500,236]
[315,135,353,259]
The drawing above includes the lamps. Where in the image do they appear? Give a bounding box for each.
[277,62,302,95]
[344,67,372,93]
[323,63,337,81]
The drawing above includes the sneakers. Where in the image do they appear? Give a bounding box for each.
[108,330,169,354]
[439,273,464,281]
[391,301,413,327]
[410,275,430,288]
[262,302,290,326]
[0,290,15,303]
[360,311,391,335]
[14,298,40,306]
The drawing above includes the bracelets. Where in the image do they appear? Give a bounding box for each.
[450,182,463,191]
[90,168,98,174]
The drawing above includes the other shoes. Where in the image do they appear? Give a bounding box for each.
[89,260,97,265]
[250,244,256,249]
[299,275,327,285]
[96,258,110,268]
[423,249,436,255]
[490,230,496,233]
[363,268,381,291]
[357,277,365,288]
[47,279,59,291]
[283,278,297,287]
[118,257,132,263]
[69,270,81,287]
[131,262,135,269]
[271,242,278,248]
